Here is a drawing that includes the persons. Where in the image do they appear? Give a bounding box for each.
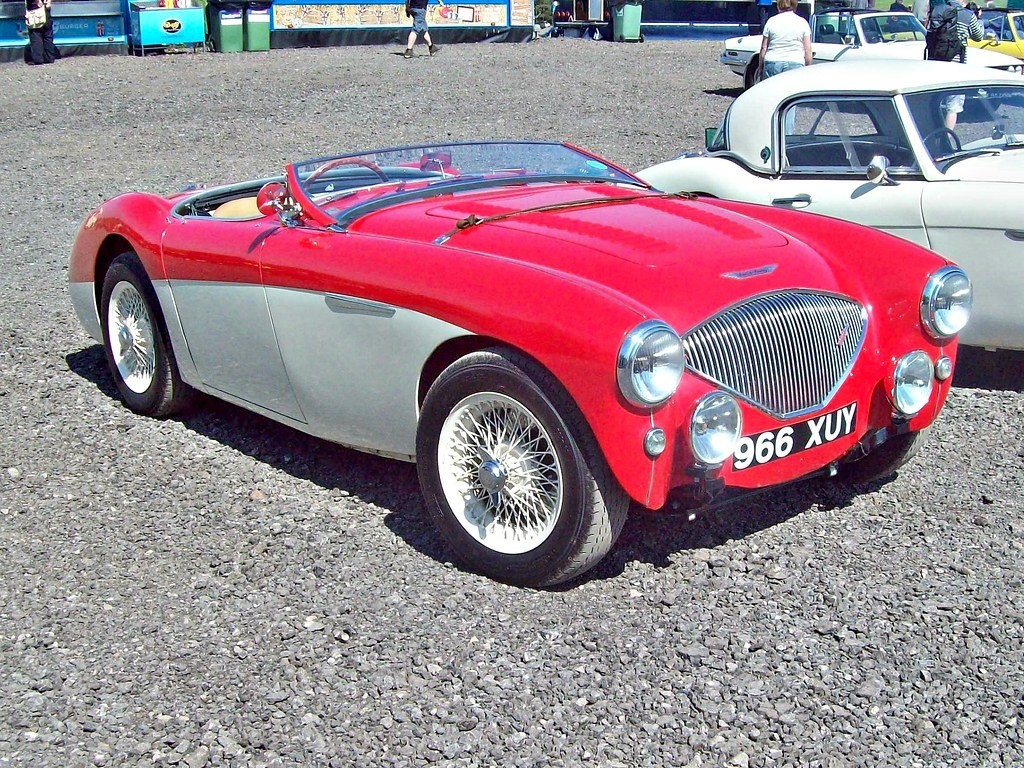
[887,0,914,32]
[925,0,984,142]
[758,0,812,139]
[984,0,996,9]
[24,0,54,65]
[404,0,443,59]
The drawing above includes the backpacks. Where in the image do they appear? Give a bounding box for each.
[924,8,961,61]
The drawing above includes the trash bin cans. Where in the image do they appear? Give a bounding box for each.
[610,0,646,44]
[207,1,243,53]
[243,8,271,51]
[128,2,206,57]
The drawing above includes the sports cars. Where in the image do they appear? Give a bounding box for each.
[610,57,1024,357]
[68,139,974,591]
[717,6,1024,90]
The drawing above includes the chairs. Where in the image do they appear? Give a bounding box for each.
[214,196,263,219]
[820,24,842,43]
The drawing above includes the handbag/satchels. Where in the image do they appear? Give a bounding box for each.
[25,6,46,29]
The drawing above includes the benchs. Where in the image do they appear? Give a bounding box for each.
[785,141,911,168]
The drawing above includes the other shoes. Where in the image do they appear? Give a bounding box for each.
[428,42,442,56]
[404,49,419,58]
